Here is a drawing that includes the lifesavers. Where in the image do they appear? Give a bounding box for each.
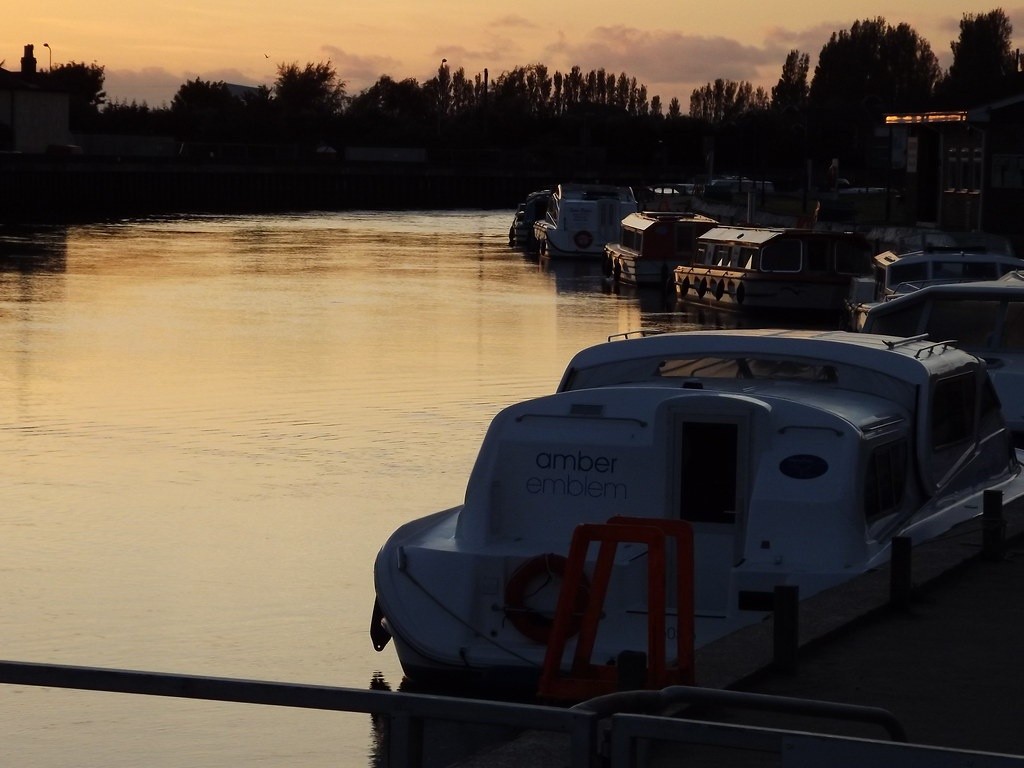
[504,552,593,645]
[574,230,593,249]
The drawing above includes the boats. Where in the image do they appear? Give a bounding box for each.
[368,319,1024,731]
[509,175,1024,434]
